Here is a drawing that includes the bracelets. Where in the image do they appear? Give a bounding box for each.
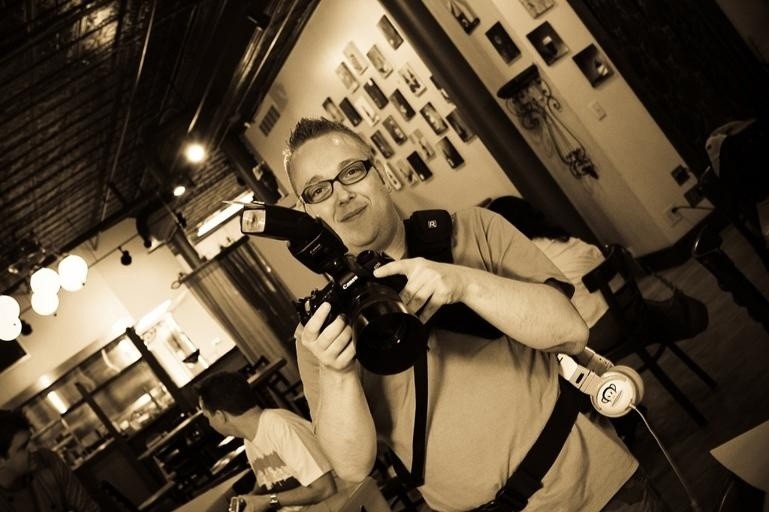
[268,491,282,510]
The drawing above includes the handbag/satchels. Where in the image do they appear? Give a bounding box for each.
[606,240,711,344]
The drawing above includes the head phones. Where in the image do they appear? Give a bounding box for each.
[557,346,645,418]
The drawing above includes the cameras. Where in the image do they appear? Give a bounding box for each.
[237,207,427,375]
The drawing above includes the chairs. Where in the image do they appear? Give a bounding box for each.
[136,121,767,510]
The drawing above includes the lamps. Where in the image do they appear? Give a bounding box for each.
[0,221,88,347]
[17,199,188,337]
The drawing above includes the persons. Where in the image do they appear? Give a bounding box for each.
[0,407,103,512]
[285,116,675,512]
[487,196,635,354]
[196,370,338,512]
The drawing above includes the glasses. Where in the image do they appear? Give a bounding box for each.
[296,159,376,209]
